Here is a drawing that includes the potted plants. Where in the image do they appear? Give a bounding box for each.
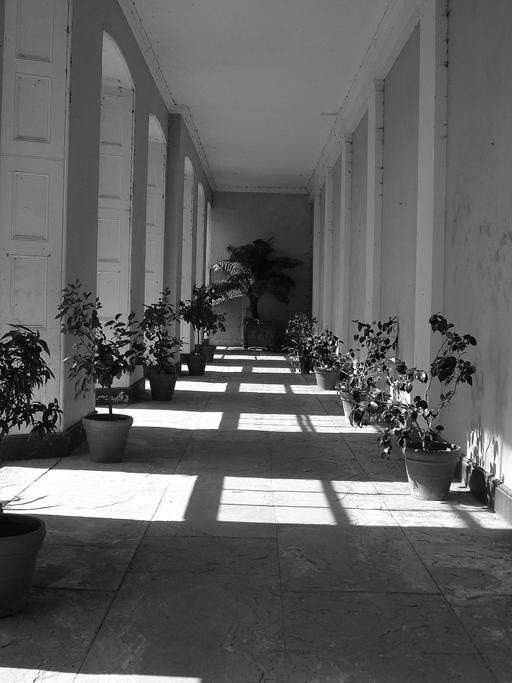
[284,311,478,499]
[177,282,227,376]
[54,277,153,463]
[139,287,188,402]
[1,319,65,621]
[209,236,303,349]
